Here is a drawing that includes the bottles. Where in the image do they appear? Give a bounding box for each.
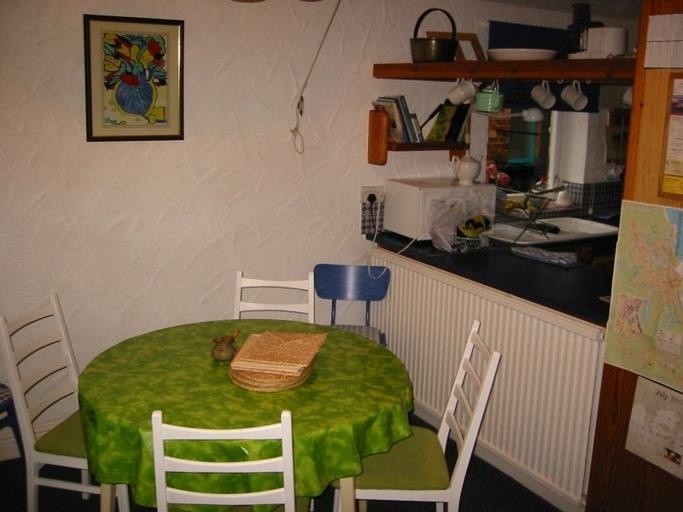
[212,336,237,361]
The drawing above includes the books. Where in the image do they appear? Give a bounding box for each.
[370,93,474,144]
[229,328,330,377]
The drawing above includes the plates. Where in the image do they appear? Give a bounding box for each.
[485,46,560,65]
[481,214,619,246]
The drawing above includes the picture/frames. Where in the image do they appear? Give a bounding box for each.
[83,14,184,141]
[657,73,683,199]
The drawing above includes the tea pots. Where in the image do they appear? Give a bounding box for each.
[446,149,487,187]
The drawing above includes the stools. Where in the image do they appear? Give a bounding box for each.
[0,383,29,475]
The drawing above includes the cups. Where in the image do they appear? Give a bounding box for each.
[446,80,635,113]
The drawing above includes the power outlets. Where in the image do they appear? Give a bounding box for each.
[360,184,385,204]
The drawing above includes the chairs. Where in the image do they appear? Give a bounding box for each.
[233,270,314,324]
[314,263,390,348]
[0,293,131,512]
[332,319,501,511]
[151,408,296,512]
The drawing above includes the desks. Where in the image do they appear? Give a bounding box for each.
[77,318,414,512]
